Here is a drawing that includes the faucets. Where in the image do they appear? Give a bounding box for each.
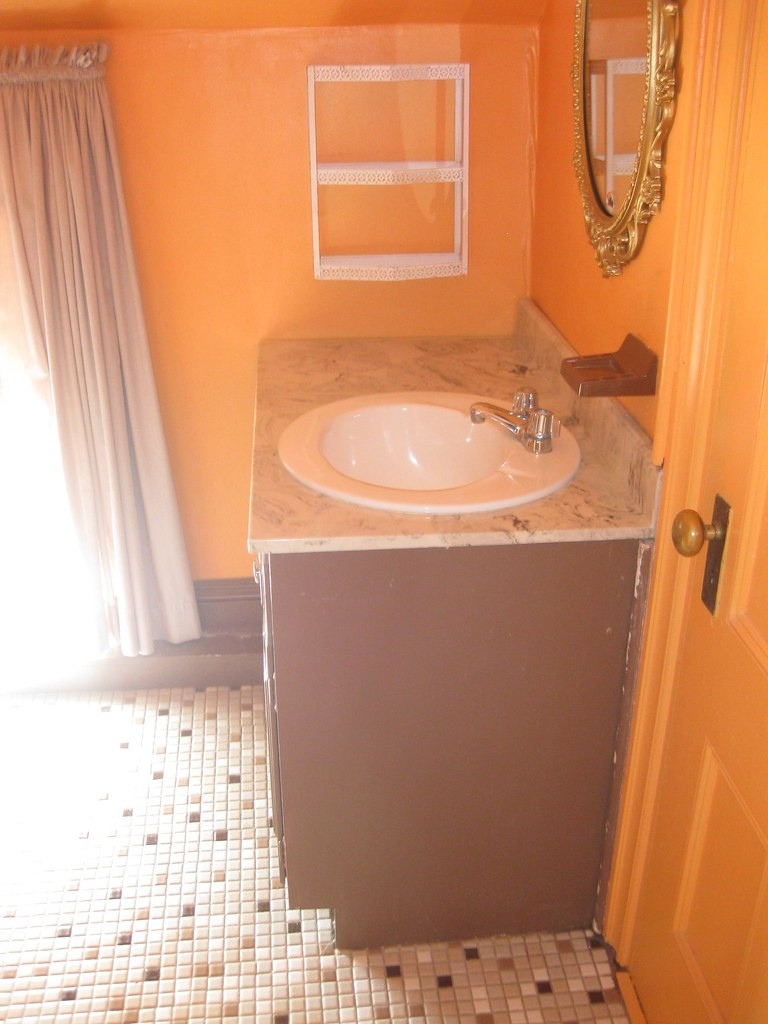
[469,401,529,437]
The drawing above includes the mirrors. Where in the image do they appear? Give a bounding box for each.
[572,0,680,278]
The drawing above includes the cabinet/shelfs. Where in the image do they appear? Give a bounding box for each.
[306,64,470,282]
[254,552,285,886]
[588,57,646,214]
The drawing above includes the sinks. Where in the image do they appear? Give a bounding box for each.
[276,389,583,515]
